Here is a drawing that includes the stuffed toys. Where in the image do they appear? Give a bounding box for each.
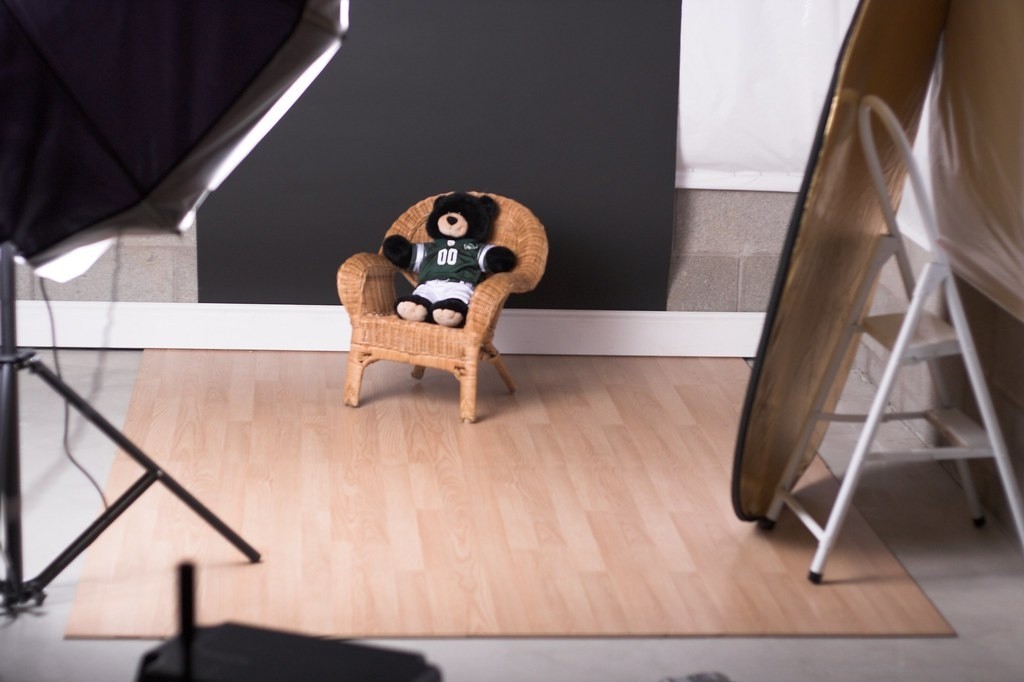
[379,191,518,328]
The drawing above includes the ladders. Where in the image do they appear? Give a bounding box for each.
[757,95,1023,584]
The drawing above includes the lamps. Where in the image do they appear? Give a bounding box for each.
[0,0,351,614]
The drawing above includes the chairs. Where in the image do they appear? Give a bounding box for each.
[336,190,549,425]
